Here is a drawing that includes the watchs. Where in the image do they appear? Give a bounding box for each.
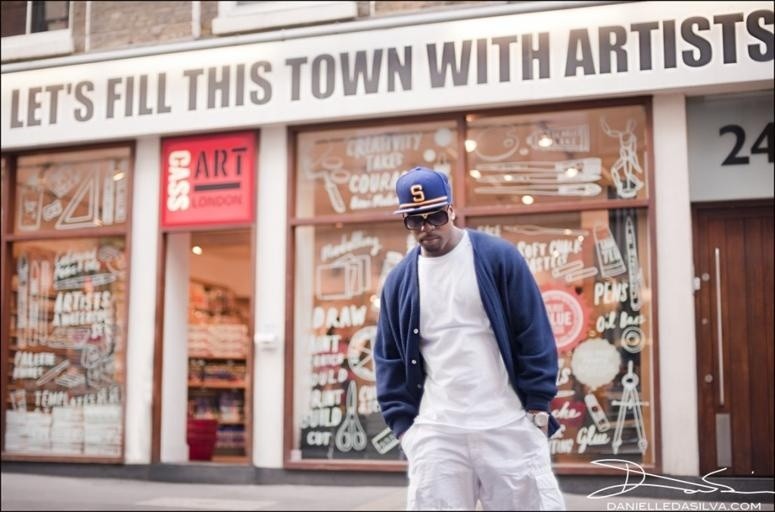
[526,411,549,428]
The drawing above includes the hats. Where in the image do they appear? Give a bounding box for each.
[393,166,453,214]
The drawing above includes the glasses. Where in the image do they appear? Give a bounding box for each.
[402,210,449,230]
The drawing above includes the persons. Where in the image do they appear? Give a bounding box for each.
[373,166,567,511]
